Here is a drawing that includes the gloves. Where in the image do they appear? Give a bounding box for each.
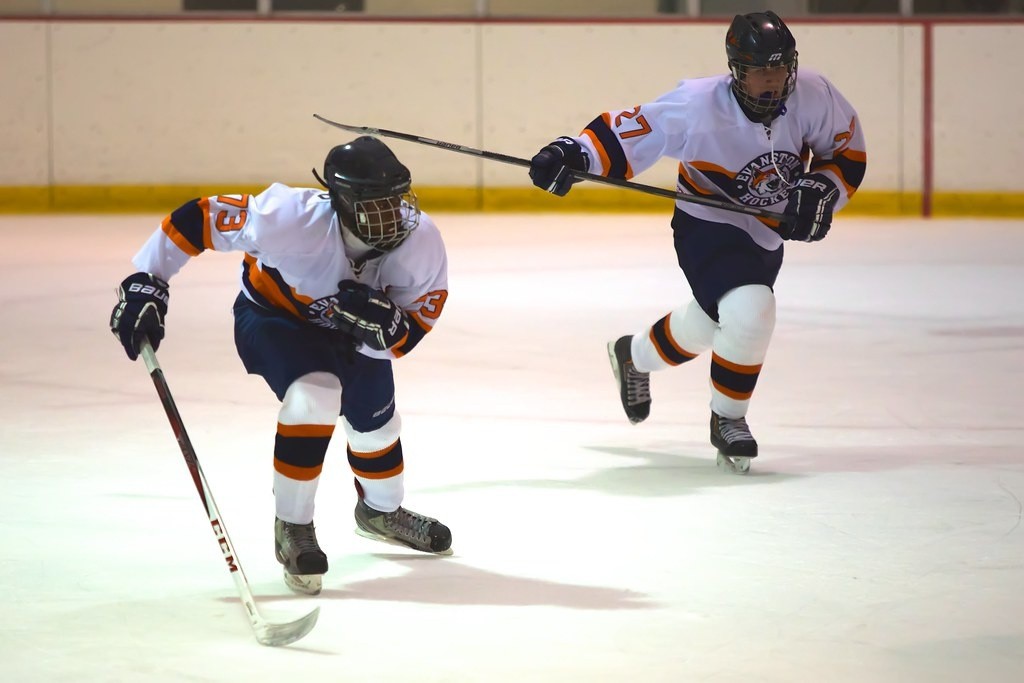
[331,278,409,352]
[528,136,591,198]
[778,173,839,242]
[110,272,170,362]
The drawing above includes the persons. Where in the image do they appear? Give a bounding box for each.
[108,136,453,594]
[529,11,867,476]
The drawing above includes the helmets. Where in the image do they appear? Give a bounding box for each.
[324,135,420,252]
[726,9,798,112]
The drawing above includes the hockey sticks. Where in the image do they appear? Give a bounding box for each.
[313,111,793,224]
[140,335,321,647]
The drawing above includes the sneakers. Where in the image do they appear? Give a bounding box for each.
[710,412,758,473]
[352,478,454,555]
[274,517,328,596]
[607,335,652,423]
